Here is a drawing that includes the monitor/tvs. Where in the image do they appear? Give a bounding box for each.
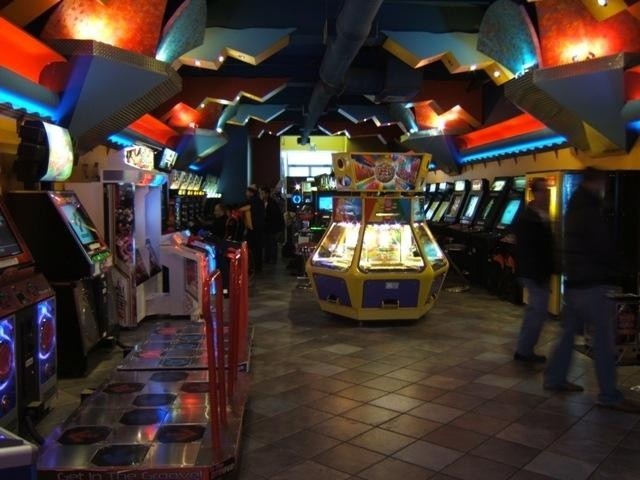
[0,206,23,259]
[61,202,97,246]
[318,196,332,213]
[423,194,521,224]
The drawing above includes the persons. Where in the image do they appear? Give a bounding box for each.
[196,184,285,261]
[514,167,640,413]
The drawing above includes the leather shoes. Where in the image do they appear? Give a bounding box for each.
[596,396,640,414]
[541,379,584,392]
[513,348,549,363]
